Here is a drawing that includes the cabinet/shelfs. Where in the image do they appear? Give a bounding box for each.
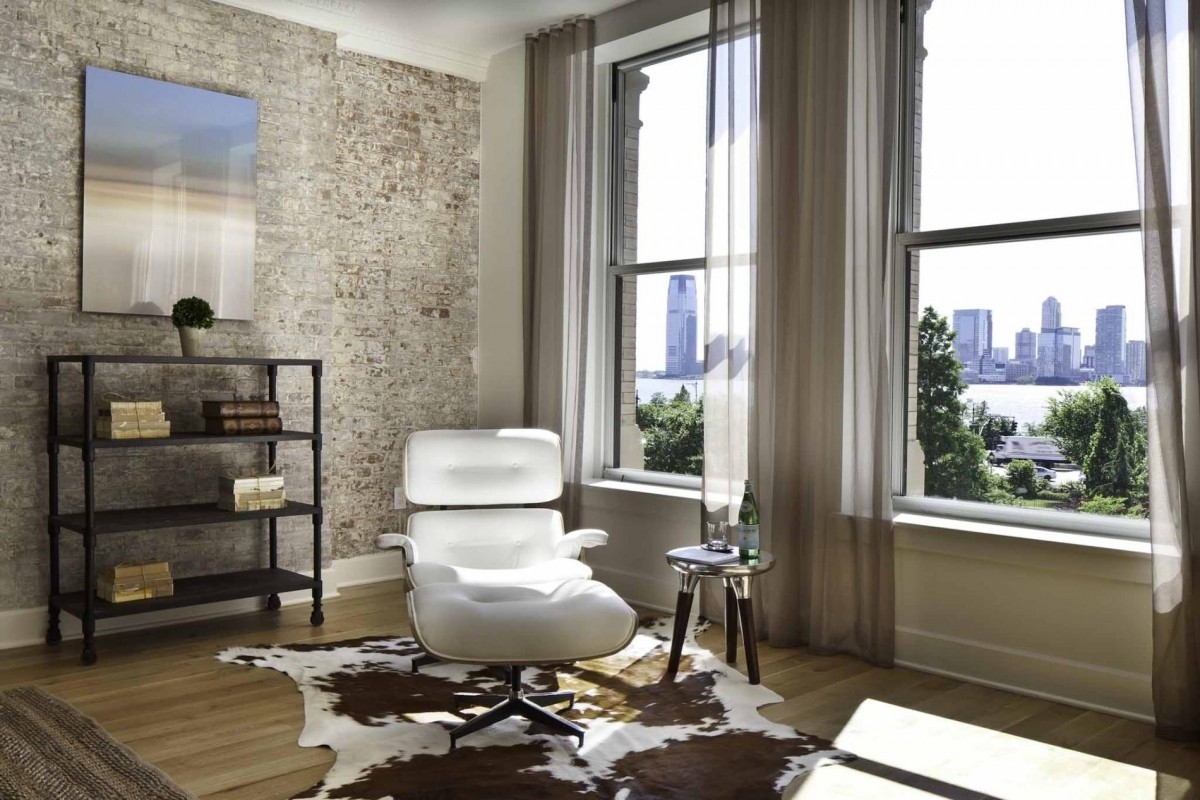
[47,354,326,665]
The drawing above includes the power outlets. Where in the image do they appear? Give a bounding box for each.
[394,487,407,509]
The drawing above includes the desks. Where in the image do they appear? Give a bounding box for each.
[667,546,777,686]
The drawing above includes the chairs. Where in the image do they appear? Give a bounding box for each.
[375,428,640,749]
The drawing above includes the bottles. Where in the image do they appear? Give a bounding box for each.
[738,480,761,558]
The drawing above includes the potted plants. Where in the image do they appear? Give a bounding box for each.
[171,297,216,358]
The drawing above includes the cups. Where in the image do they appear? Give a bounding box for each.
[706,520,730,550]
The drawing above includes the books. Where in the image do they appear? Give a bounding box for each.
[96,560,174,603]
[219,473,296,512]
[98,400,171,439]
[201,399,284,436]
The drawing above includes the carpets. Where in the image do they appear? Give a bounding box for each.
[0,685,197,800]
[213,614,857,800]
